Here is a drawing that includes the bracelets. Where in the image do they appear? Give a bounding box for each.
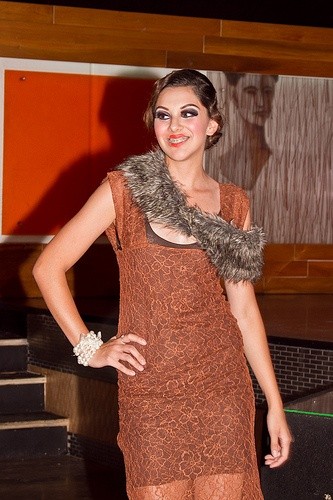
[72,330,104,367]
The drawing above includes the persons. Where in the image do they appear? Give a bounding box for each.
[32,68,292,500]
[217,70,278,196]
